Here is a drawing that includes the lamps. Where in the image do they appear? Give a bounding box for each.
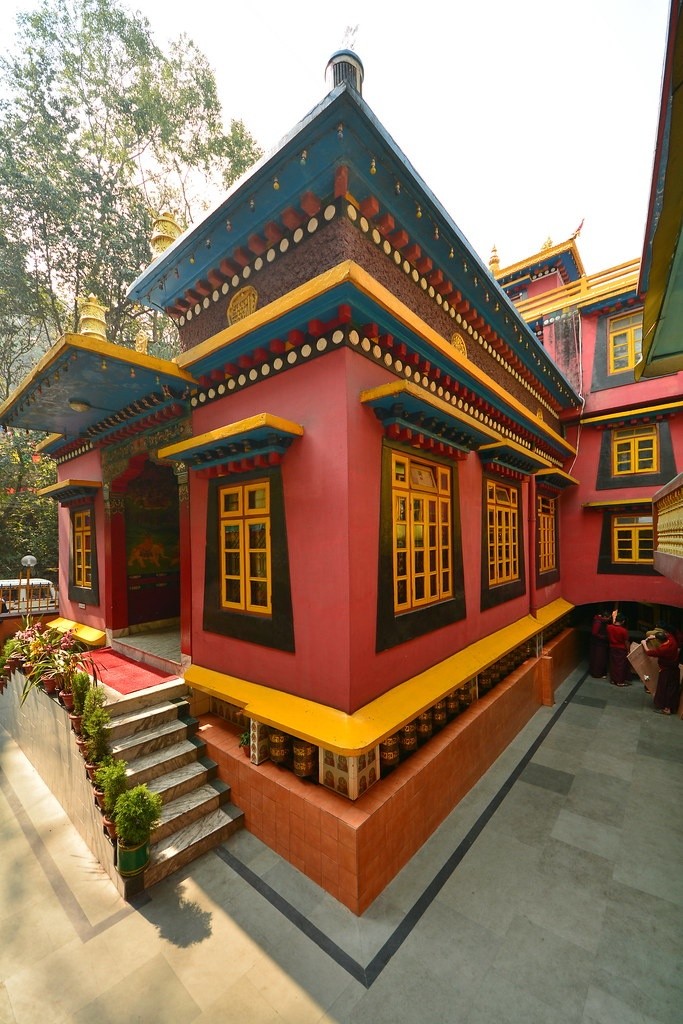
[69,401,89,412]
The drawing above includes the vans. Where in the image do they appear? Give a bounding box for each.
[0,578,59,612]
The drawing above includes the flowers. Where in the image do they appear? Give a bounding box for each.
[18,622,80,679]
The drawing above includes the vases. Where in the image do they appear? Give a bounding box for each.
[60,688,74,710]
[55,688,65,705]
[22,662,35,679]
[40,673,57,693]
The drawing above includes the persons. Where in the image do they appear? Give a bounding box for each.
[636,626,680,715]
[581,610,630,686]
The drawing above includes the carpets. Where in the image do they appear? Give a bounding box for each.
[70,646,179,694]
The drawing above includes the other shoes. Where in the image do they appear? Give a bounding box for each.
[645,689,650,692]
[617,682,629,686]
[659,709,672,715]
[611,680,617,684]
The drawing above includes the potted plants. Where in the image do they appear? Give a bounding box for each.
[113,783,163,877]
[76,685,107,759]
[0,635,31,693]
[92,754,118,810]
[84,708,115,781]
[99,757,130,840]
[69,671,92,733]
[237,730,250,758]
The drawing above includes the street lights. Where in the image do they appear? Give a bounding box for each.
[21,549,37,600]
